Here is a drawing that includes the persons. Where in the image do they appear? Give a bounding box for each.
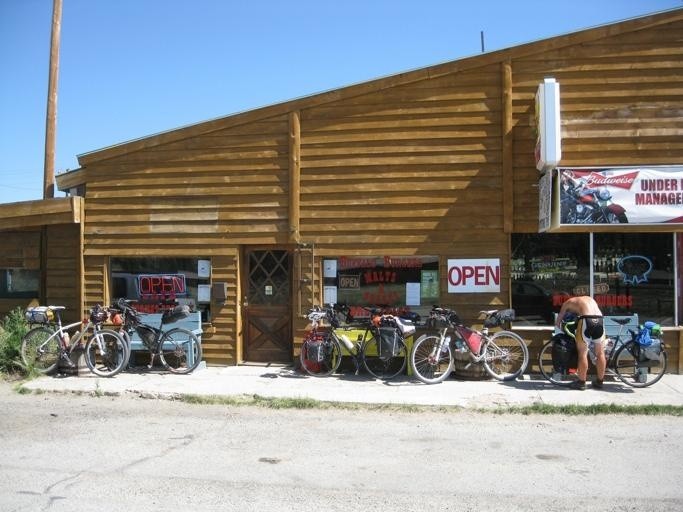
[553,294,607,390]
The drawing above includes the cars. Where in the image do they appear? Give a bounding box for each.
[511,278,566,322]
[111,271,189,296]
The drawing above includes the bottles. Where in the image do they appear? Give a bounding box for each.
[441,335,451,353]
[116,328,125,346]
[69,330,80,346]
[340,335,353,350]
[63,330,69,348]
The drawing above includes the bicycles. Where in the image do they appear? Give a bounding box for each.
[298,303,408,381]
[410,305,529,385]
[106,296,202,377]
[538,315,669,388]
[19,302,128,376]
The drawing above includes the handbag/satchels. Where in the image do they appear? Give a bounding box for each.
[373,326,400,360]
[637,338,662,367]
[552,334,578,370]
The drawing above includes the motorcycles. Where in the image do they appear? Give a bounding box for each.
[560,168,630,224]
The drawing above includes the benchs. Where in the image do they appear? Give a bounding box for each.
[114,310,206,374]
[329,328,414,376]
[551,313,642,376]
